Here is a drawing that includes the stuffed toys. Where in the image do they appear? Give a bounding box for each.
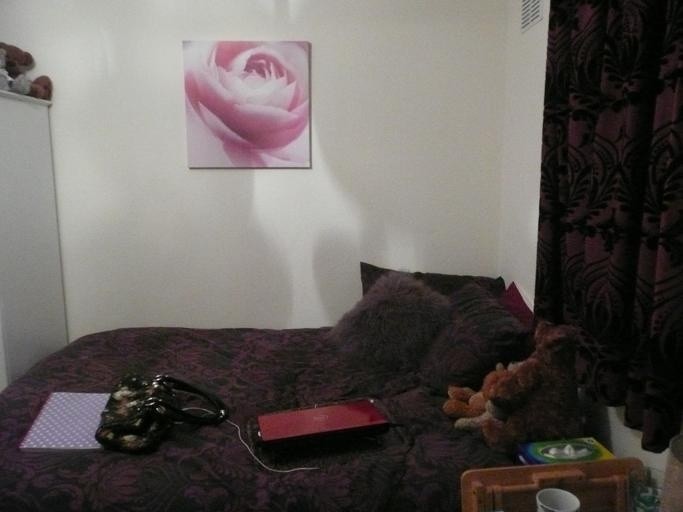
[0,43,52,101]
[453,360,525,430]
[480,320,599,456]
[441,369,512,417]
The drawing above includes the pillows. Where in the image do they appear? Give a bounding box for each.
[324,261,535,396]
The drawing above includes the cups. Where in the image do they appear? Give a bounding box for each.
[534,488,582,512]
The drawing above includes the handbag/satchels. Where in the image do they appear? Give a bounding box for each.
[95,373,175,454]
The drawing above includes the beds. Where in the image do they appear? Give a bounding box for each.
[3,327,523,509]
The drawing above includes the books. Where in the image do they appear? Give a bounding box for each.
[14,390,111,455]
